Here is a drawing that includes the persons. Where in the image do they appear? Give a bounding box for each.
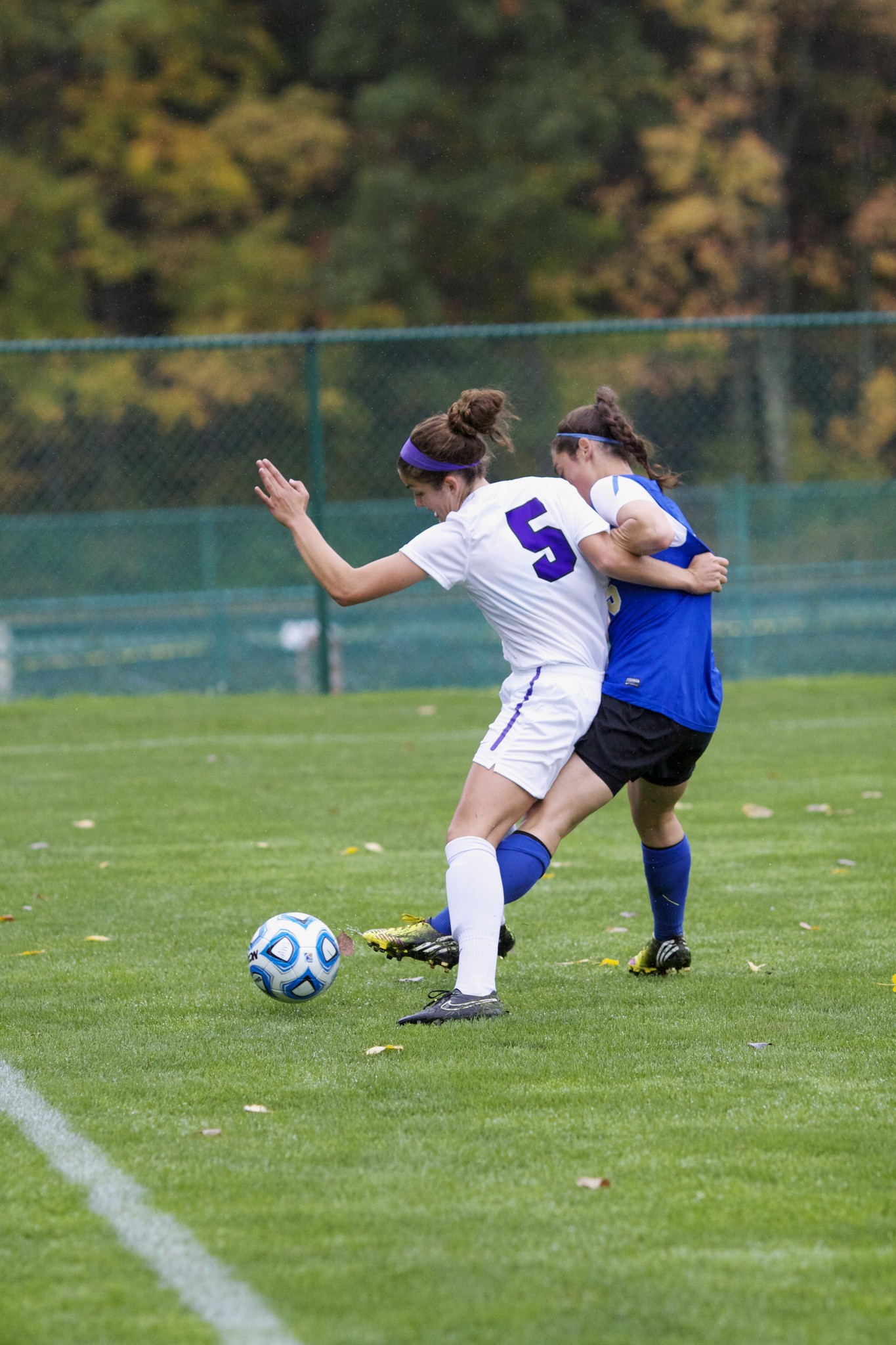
[363,384,720,978]
[255,384,729,1024]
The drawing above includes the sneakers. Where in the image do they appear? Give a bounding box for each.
[398,988,505,1024]
[498,923,515,958]
[626,932,691,975]
[361,913,459,972]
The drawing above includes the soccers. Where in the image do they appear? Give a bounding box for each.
[249,913,340,1004]
